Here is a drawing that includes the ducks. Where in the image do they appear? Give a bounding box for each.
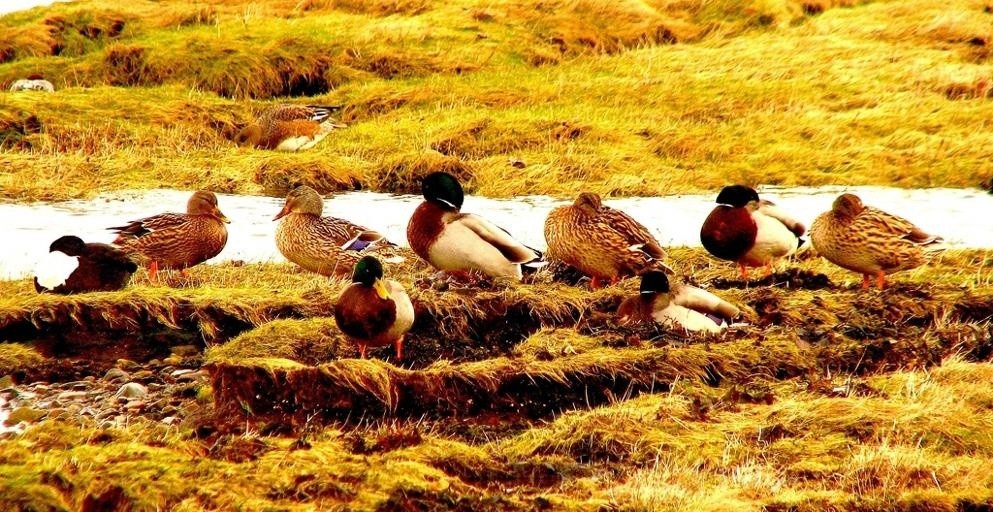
[810,192,948,290]
[35,234,136,293]
[270,183,405,276]
[542,191,678,289]
[235,99,348,155]
[108,188,235,282]
[331,253,419,362]
[698,182,807,282]
[612,265,748,335]
[404,170,537,287]
